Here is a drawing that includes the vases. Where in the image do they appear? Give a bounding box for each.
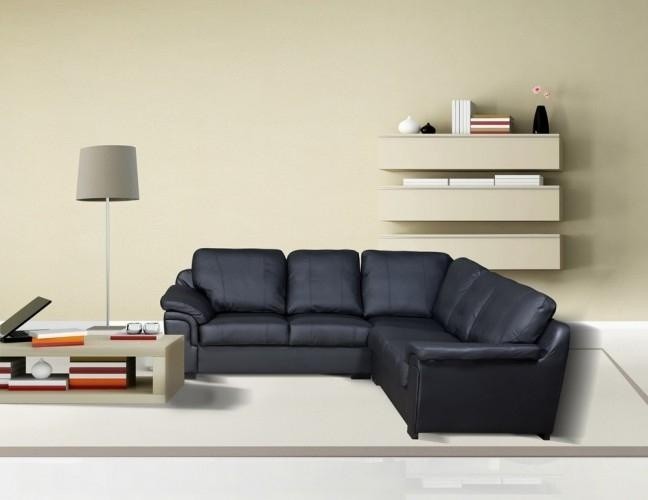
[532,105,550,134]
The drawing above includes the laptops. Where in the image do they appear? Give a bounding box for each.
[0,296,52,342]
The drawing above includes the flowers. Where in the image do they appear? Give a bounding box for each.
[531,85,554,105]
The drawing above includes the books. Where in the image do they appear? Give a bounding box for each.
[29,328,87,347]
[452,99,512,134]
[403,173,543,188]
[110,331,163,340]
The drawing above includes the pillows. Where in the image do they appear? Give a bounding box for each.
[287,250,363,317]
[192,248,288,316]
[432,257,556,345]
[361,250,453,320]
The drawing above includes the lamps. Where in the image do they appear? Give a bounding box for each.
[77,145,140,331]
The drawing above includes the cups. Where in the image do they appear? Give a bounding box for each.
[143,322,161,335]
[126,322,143,334]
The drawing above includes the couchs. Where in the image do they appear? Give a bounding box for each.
[160,247,570,442]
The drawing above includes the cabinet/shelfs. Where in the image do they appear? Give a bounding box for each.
[380,133,565,272]
